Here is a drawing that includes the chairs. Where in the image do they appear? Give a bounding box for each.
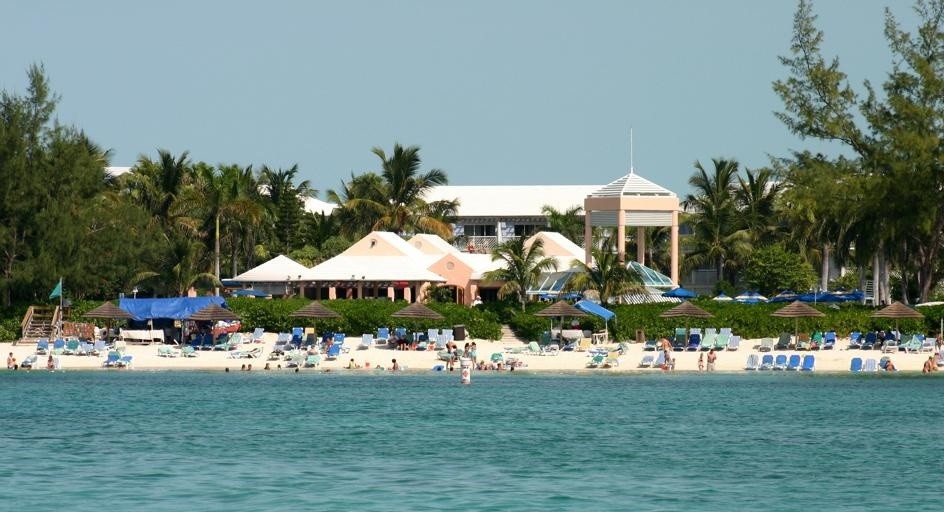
[269,326,345,369]
[525,330,624,369]
[759,331,837,352]
[643,352,670,368]
[850,329,940,352]
[670,329,742,350]
[36,335,133,370]
[850,357,891,371]
[359,327,464,362]
[746,354,815,370]
[157,327,264,359]
[643,340,664,352]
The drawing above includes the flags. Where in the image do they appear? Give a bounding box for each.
[48,280,62,300]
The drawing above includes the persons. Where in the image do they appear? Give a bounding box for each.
[854,331,944,375]
[801,324,822,350]
[5,325,124,373]
[778,328,793,350]
[658,335,718,374]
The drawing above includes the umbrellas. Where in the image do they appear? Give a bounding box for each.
[711,287,869,303]
[539,286,583,302]
[660,287,700,302]
